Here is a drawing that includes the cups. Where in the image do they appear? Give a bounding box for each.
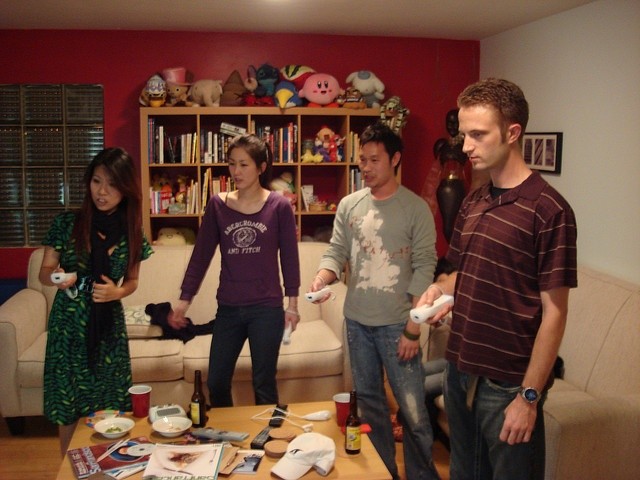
[332,392,350,427]
[126,383,153,418]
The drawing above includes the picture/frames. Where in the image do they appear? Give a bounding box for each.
[521,131,563,174]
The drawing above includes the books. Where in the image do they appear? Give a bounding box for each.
[202,167,237,213]
[103,460,149,479]
[230,449,265,474]
[150,187,172,215]
[148,117,298,164]
[187,179,199,214]
[66,436,154,480]
[142,442,224,480]
[349,130,367,193]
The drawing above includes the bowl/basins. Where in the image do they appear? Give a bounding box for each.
[151,416,192,438]
[93,417,135,440]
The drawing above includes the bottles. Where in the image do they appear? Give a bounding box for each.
[344,389,362,455]
[190,370,206,428]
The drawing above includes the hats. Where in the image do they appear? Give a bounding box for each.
[271,432,336,479]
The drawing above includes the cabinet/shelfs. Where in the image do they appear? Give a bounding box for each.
[138,105,403,247]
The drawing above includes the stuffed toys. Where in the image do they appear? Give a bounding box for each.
[298,73,345,107]
[279,64,317,92]
[301,124,346,162]
[138,74,168,107]
[342,88,368,109]
[345,71,385,109]
[186,79,223,107]
[270,169,297,212]
[244,76,275,106]
[247,64,280,98]
[220,70,247,106]
[153,168,190,202]
[273,79,309,109]
[379,96,410,136]
[161,67,192,107]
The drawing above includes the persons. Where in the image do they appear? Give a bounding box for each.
[306,123,438,480]
[415,78,578,480]
[419,110,470,246]
[166,134,301,408]
[38,147,155,459]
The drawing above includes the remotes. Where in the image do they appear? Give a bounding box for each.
[251,427,273,449]
[269,404,288,427]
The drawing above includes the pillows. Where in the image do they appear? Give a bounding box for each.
[122,303,162,338]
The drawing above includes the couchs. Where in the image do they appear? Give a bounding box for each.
[415,266,639,480]
[1,242,356,429]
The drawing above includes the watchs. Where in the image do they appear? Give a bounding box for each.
[517,385,542,404]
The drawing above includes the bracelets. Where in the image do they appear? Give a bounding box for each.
[284,309,300,316]
[316,275,327,286]
[289,304,298,307]
[53,269,57,273]
[403,329,421,340]
